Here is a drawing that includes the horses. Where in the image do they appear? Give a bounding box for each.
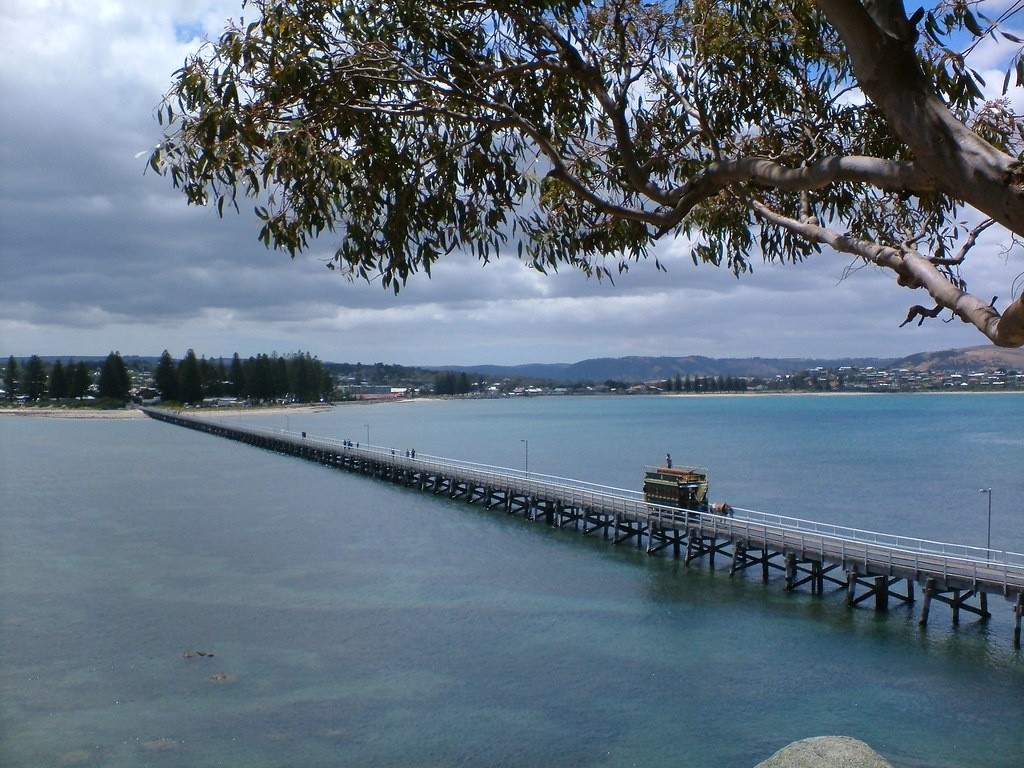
[710,502,735,525]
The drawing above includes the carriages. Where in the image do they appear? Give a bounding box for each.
[642,464,734,520]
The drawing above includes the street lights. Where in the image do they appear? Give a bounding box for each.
[978,487,993,567]
[363,424,369,447]
[521,439,528,479]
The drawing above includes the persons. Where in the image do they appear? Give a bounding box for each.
[411,449,415,458]
[391,448,395,457]
[666,453,672,468]
[406,449,409,458]
[357,441,359,449]
[342,438,353,451]
